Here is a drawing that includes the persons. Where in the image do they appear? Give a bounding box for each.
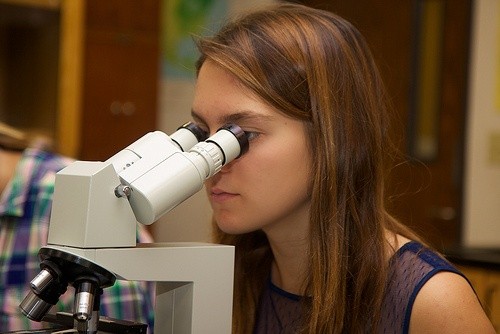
[0,143,157,334]
[188,3,498,333]
